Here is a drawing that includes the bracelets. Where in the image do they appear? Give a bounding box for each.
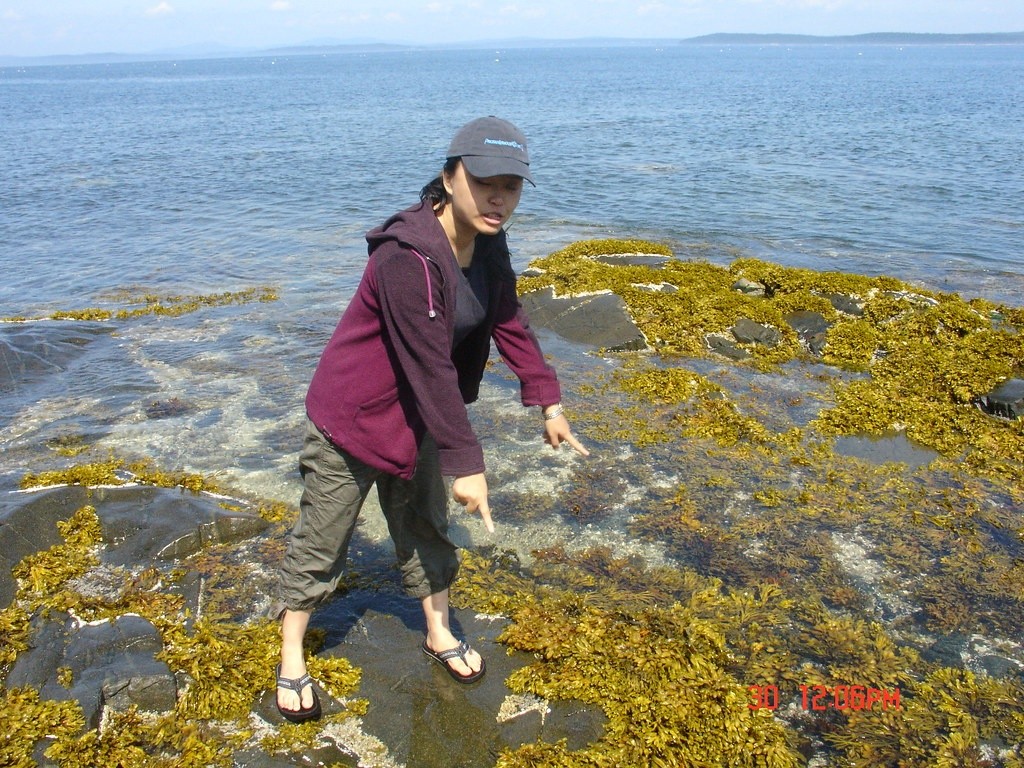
[542,406,563,420]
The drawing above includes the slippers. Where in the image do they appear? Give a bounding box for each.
[276,663,320,720]
[422,639,486,684]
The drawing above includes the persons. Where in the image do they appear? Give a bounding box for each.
[273,115,589,722]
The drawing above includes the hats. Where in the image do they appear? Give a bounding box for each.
[446,115,536,187]
